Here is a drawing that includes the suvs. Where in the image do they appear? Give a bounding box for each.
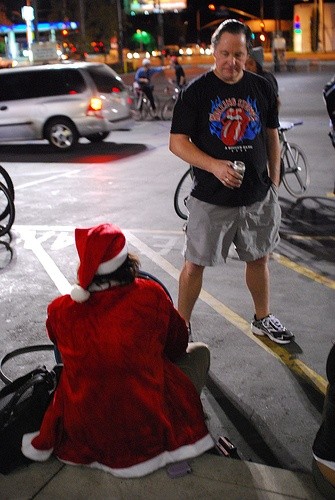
[0,60,137,154]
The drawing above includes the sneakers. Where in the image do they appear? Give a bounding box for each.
[251,314,295,345]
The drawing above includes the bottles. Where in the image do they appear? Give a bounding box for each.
[232,160,246,185]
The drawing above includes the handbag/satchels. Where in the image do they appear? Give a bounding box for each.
[0,345,63,475]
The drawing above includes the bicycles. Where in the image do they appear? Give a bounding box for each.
[128,78,160,121]
[161,78,184,122]
[174,121,311,220]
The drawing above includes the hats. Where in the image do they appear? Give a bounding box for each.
[71,223,128,301]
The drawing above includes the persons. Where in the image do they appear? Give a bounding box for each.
[272,31,287,72]
[133,58,171,114]
[313,343,335,487]
[241,45,282,118]
[171,56,187,90]
[20,224,212,479]
[169,20,296,344]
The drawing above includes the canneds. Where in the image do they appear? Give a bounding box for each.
[232,160,246,182]
